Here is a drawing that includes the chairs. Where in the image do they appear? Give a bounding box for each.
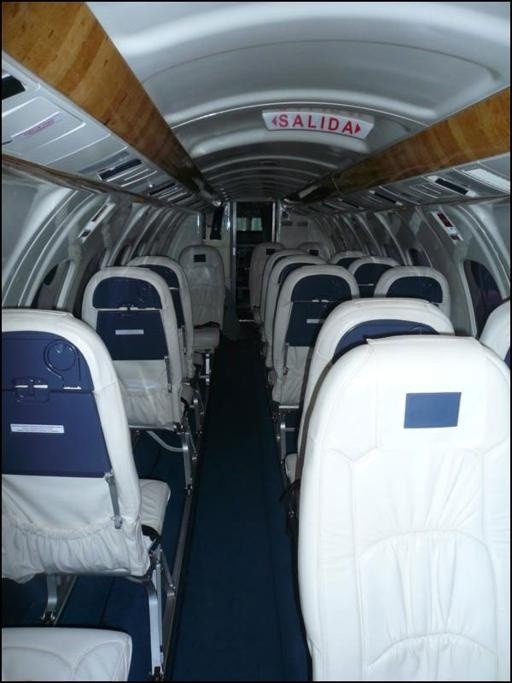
[268,265,360,406]
[373,265,449,305]
[80,269,192,431]
[329,251,364,266]
[480,301,510,360]
[178,244,224,346]
[0,629,133,678]
[299,243,325,259]
[249,241,286,310]
[283,297,453,485]
[255,248,308,327]
[1,307,168,582]
[264,254,325,367]
[298,334,511,678]
[128,255,195,375]
